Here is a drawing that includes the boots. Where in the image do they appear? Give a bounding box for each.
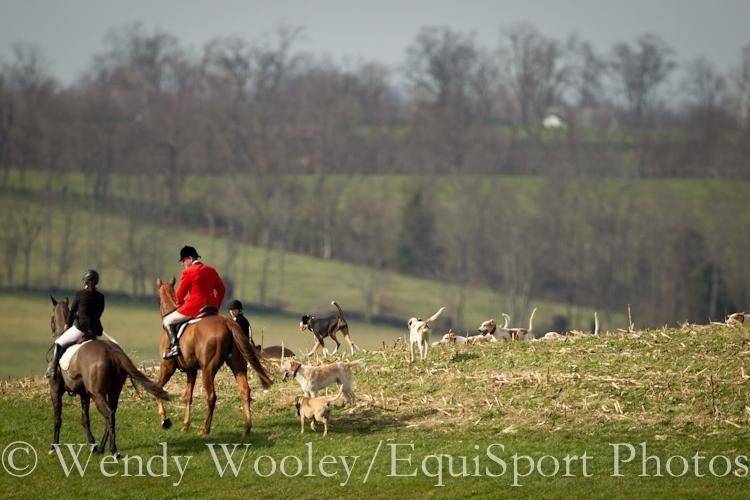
[166,324,180,357]
[46,343,62,377]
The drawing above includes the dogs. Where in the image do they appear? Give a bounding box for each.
[278,359,363,408]
[540,311,600,340]
[725,312,749,326]
[408,305,447,365]
[476,307,538,341]
[290,384,346,438]
[298,300,354,356]
[439,332,490,345]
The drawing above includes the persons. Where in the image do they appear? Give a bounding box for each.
[45,269,105,379]
[227,299,250,340]
[162,245,226,360]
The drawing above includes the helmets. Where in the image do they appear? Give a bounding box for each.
[84,269,98,284]
[179,246,199,262]
[229,300,242,309]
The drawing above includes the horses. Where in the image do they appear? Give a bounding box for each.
[44,294,170,460]
[153,275,276,442]
